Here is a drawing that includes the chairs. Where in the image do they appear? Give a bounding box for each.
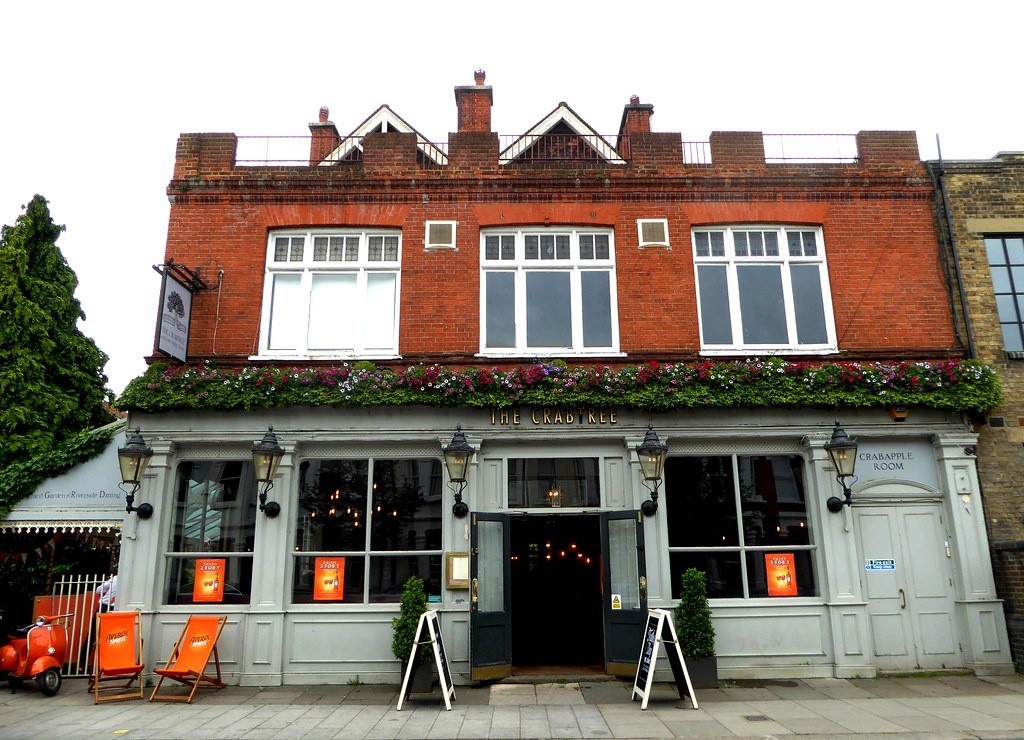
[149,615,228,703]
[88,608,143,704]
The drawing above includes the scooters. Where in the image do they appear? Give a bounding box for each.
[0,608,76,696]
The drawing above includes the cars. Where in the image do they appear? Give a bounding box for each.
[165,578,245,600]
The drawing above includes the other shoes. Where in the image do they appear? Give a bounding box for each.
[79,666,84,671]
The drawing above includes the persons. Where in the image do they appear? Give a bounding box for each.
[77,574,118,674]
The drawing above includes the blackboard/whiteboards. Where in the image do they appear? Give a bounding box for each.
[633,609,665,699]
[427,609,454,698]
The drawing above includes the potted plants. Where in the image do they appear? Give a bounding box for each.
[674,568,719,689]
[392,575,436,693]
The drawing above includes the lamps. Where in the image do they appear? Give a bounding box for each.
[251,424,286,518]
[441,422,475,520]
[117,425,153,520]
[635,420,668,517]
[823,418,858,513]
